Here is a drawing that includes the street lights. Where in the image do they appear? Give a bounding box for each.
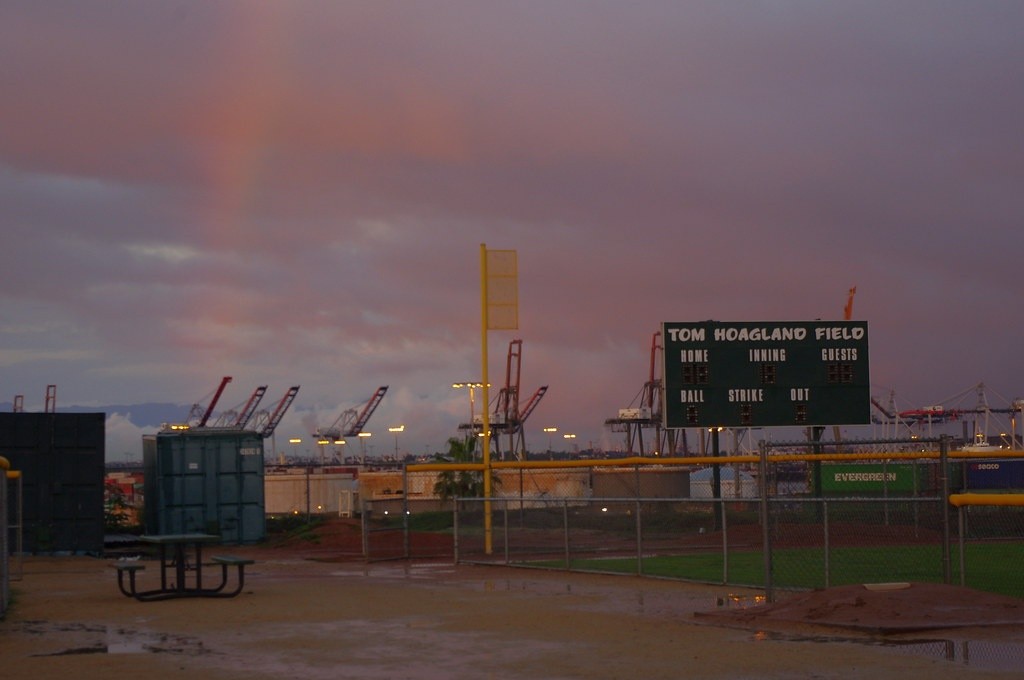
[543,427,558,457]
[452,381,492,463]
[358,432,372,465]
[289,439,301,466]
[388,426,405,470]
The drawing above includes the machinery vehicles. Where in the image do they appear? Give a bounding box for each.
[176,373,300,440]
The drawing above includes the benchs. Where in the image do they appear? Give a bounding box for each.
[109,555,254,599]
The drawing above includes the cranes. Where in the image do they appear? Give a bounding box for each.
[603,329,713,459]
[456,337,551,464]
[311,385,390,472]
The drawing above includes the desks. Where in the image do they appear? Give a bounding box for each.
[141,534,219,599]
